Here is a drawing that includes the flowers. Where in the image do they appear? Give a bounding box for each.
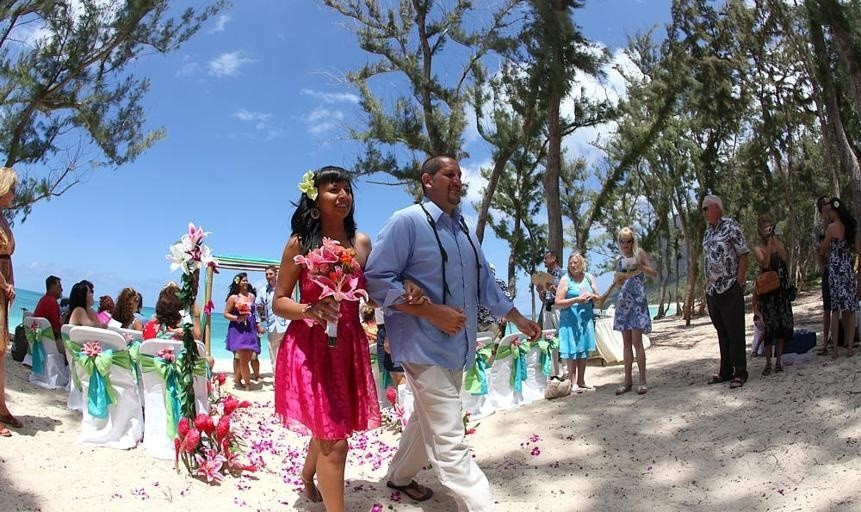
[164,222,219,275]
[297,167,319,203]
[294,236,371,348]
[236,302,252,324]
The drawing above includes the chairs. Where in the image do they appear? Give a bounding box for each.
[22,300,214,460]
[368,327,563,432]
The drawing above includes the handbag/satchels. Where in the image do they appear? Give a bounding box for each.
[755,270,781,297]
[545,378,572,399]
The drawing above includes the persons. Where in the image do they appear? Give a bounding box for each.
[223,263,511,389]
[537,250,566,377]
[366,156,543,512]
[0,166,23,436]
[31,276,215,388]
[753,217,793,376]
[751,315,765,356]
[614,228,657,395]
[701,195,750,387]
[554,254,601,389]
[820,197,858,360]
[272,167,432,512]
[813,195,860,357]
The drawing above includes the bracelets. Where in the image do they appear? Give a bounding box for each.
[301,304,318,328]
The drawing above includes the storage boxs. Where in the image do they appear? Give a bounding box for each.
[774,332,815,354]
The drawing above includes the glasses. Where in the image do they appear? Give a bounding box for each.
[618,238,632,244]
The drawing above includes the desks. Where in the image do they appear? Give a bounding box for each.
[588,316,650,368]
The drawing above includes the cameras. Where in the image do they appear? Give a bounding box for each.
[765,226,775,235]
[544,298,555,311]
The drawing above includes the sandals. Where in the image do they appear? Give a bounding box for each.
[615,386,631,395]
[730,371,747,388]
[707,374,732,383]
[776,364,782,372]
[387,478,433,502]
[299,471,323,503]
[637,383,647,394]
[763,364,770,375]
[1,412,23,437]
